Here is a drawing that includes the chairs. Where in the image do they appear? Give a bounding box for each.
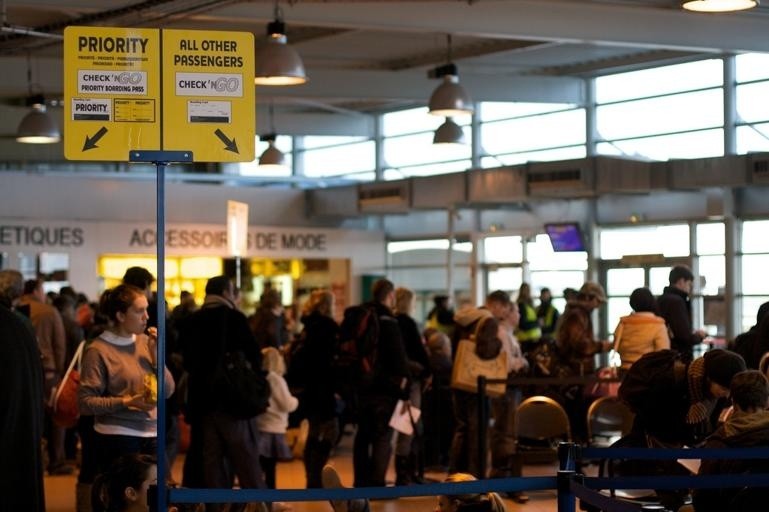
[583,390,637,499]
[507,392,579,503]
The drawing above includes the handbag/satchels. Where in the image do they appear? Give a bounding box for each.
[211,369,271,422]
[450,338,510,399]
[51,339,87,430]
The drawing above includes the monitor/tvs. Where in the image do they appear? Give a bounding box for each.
[544,219,583,252]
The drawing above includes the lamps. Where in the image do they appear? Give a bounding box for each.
[11,25,64,146]
[256,96,287,168]
[252,0,309,89]
[430,111,468,148]
[426,28,476,117]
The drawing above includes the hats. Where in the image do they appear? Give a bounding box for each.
[566,283,608,305]
[704,349,748,390]
